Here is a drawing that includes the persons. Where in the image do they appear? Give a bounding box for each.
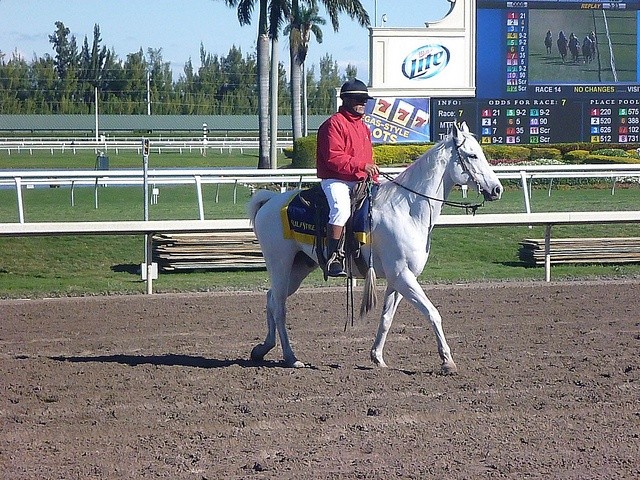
[315,78,378,274]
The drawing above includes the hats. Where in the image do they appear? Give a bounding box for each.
[340,78,373,116]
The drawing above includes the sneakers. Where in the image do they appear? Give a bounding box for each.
[327,258,343,274]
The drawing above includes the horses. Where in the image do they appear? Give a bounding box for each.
[247,119,504,377]
